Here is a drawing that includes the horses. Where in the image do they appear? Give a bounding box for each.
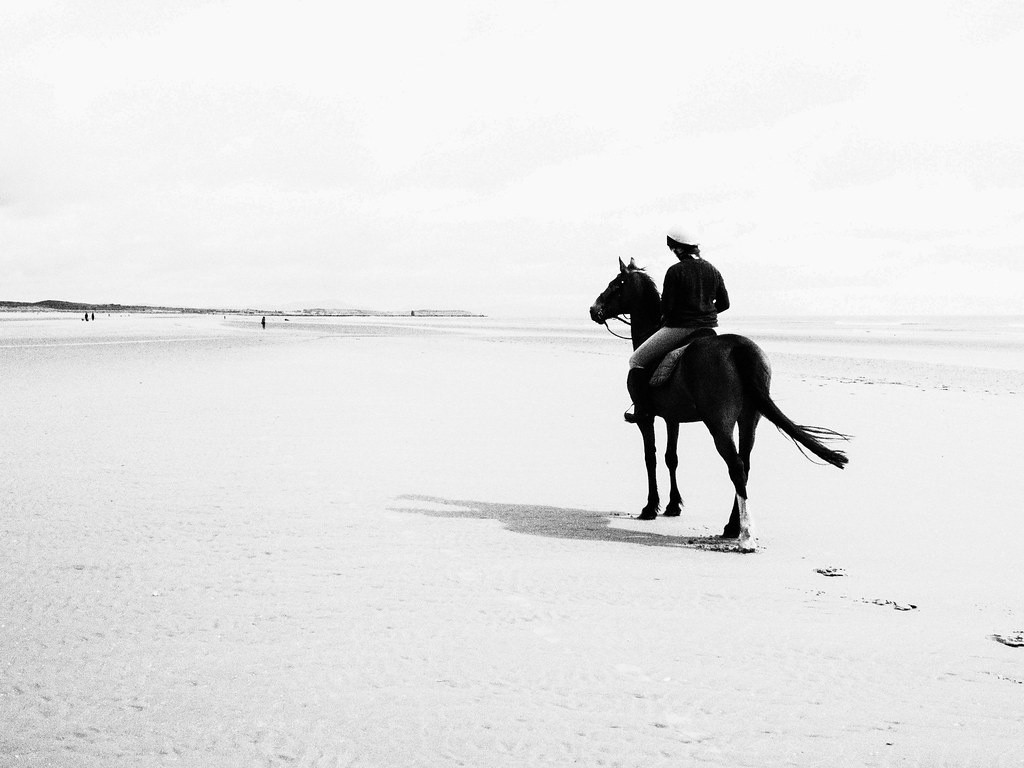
[589,254,855,551]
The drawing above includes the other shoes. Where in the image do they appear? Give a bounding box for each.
[623,411,649,423]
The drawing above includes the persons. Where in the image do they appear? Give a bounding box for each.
[625,223,730,424]
[92,311,94,321]
[261,317,265,329]
[85,313,88,321]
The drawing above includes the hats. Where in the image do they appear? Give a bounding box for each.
[666,235,700,257]
